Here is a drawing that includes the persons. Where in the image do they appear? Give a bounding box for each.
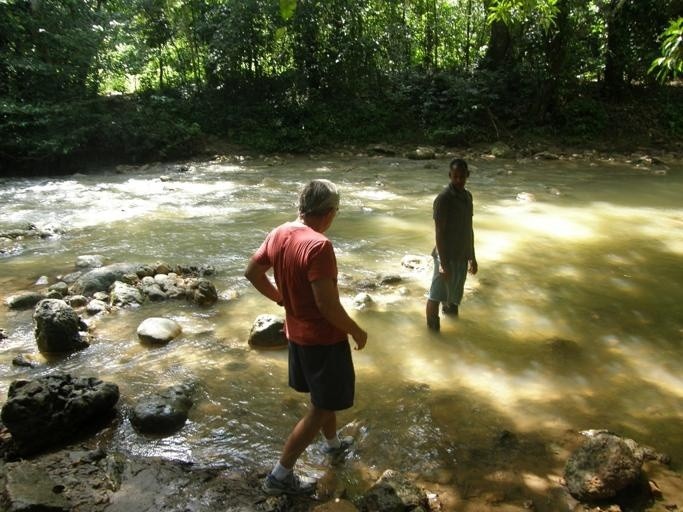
[245,179,367,495]
[426,160,477,332]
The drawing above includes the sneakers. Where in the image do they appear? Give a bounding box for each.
[262,470,318,494]
[321,436,353,456]
